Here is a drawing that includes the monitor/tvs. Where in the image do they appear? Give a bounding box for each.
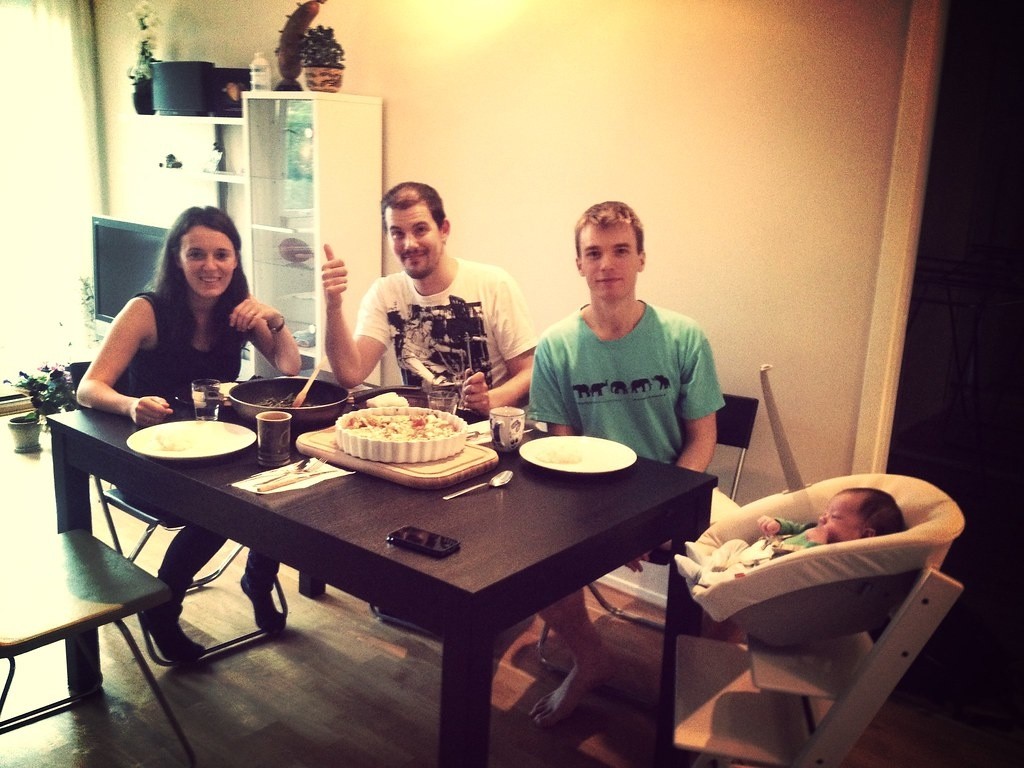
[91,215,170,324]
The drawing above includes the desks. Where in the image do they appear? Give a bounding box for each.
[52,390,720,768]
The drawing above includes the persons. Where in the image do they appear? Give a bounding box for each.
[322,181,538,412]
[675,488,906,588]
[529,201,739,726]
[77,205,301,663]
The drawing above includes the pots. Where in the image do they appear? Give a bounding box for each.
[229,377,423,434]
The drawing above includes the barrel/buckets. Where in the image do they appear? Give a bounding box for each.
[149,61,250,117]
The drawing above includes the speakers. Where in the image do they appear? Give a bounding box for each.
[150,61,218,115]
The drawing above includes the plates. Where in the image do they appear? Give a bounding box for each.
[126,420,257,458]
[174,380,246,407]
[519,435,638,474]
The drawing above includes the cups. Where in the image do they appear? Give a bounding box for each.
[255,410,292,461]
[8,416,40,453]
[191,378,221,420]
[489,406,525,453]
[428,390,459,416]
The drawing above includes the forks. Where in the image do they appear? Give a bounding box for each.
[257,456,331,492]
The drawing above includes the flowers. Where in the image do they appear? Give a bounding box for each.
[130,3,166,85]
[3,361,74,411]
[302,27,343,67]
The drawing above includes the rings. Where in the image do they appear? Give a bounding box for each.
[249,311,254,315]
[147,417,150,423]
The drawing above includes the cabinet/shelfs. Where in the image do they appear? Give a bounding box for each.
[132,113,246,183]
[243,89,384,388]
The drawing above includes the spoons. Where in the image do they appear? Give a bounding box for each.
[442,470,514,500]
[252,456,311,488]
[466,430,490,440]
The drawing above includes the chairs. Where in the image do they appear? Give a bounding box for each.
[539,391,766,659]
[68,360,286,665]
[0,529,193,758]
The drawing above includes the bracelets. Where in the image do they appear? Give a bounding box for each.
[267,315,284,334]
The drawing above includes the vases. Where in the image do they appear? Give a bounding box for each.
[38,410,62,428]
[132,78,155,112]
[304,64,345,90]
[8,416,43,454]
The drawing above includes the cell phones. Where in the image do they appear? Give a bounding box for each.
[387,525,461,558]
